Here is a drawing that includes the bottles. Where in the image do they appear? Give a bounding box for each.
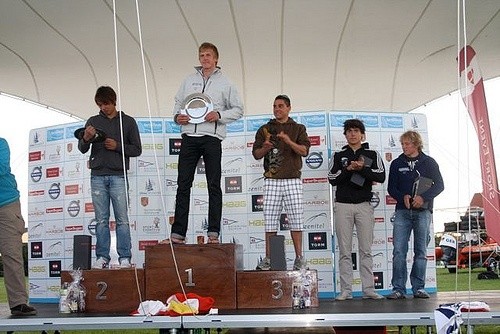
[292,286,300,310]
[303,285,310,309]
[58,281,86,314]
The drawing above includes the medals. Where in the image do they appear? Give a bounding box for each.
[272,148,279,154]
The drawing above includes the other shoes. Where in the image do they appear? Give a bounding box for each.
[10,304,36,315]
[414,290,430,298]
[387,291,407,298]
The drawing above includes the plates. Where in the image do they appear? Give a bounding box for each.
[74,128,107,143]
[180,93,213,124]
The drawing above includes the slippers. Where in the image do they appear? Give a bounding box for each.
[160,238,184,244]
[208,238,219,244]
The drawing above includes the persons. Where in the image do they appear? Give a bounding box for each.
[386,131,444,299]
[252,95,311,271]
[328,119,387,301]
[78,86,142,270]
[159,42,244,244]
[0,137,36,316]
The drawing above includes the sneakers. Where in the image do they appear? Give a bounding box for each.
[335,292,353,300]
[293,258,309,271]
[362,292,385,300]
[93,257,109,270]
[256,257,270,271]
[120,259,131,268]
